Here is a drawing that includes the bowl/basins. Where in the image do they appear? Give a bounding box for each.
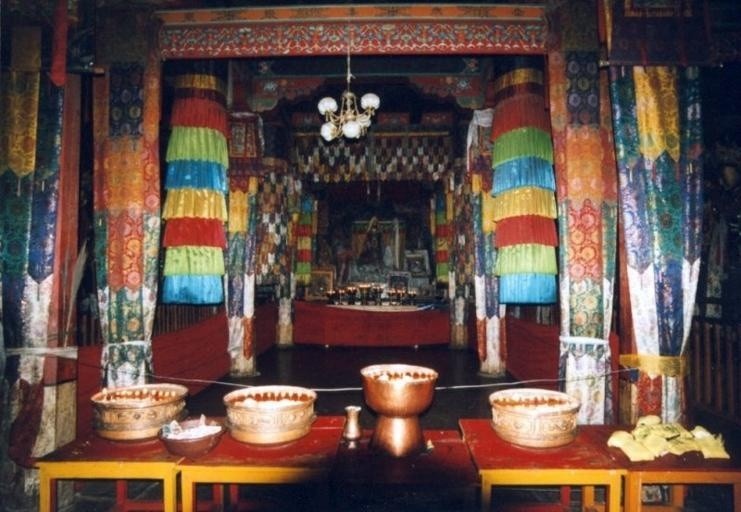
[89,384,190,442]
[489,389,583,449]
[152,420,227,460]
[220,386,317,446]
[361,365,439,462]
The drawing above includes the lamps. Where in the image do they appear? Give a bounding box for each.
[317,16,382,140]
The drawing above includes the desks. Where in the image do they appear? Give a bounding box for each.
[33,414,228,512]
[458,412,626,512]
[175,414,345,512]
[577,425,740,511]
[341,428,482,512]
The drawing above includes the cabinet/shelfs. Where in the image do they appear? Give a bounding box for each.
[291,295,449,352]
